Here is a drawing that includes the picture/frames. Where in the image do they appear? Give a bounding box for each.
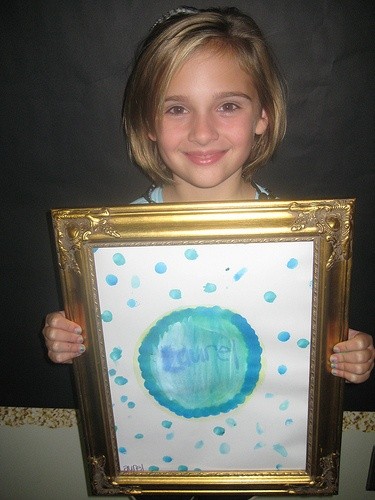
[48,198,356,498]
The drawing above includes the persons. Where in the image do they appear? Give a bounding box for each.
[41,5,375,387]
